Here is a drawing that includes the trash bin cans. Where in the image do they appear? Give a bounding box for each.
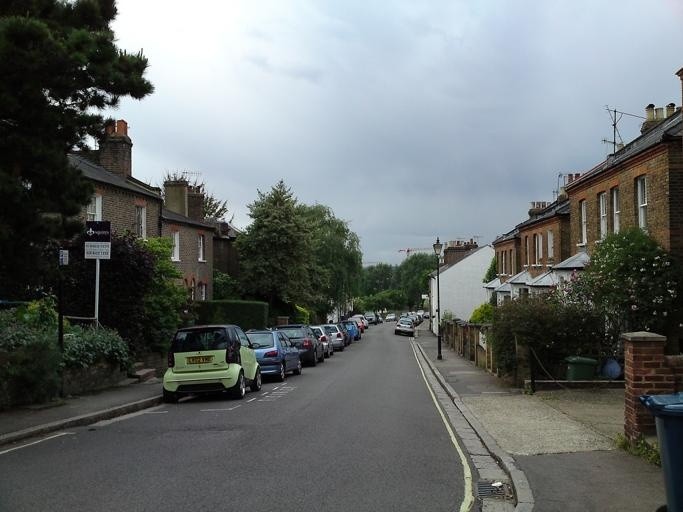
[558,356,598,381]
[638,392,683,512]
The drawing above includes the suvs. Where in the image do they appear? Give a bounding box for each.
[270,323,326,367]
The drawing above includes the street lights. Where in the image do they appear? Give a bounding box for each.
[431,235,445,360]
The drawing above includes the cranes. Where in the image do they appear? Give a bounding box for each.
[398,246,431,258]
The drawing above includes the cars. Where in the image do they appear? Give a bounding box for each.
[240,325,305,383]
[161,322,263,402]
[310,308,430,361]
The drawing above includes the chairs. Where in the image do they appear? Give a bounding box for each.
[186,332,206,351]
[208,332,225,350]
